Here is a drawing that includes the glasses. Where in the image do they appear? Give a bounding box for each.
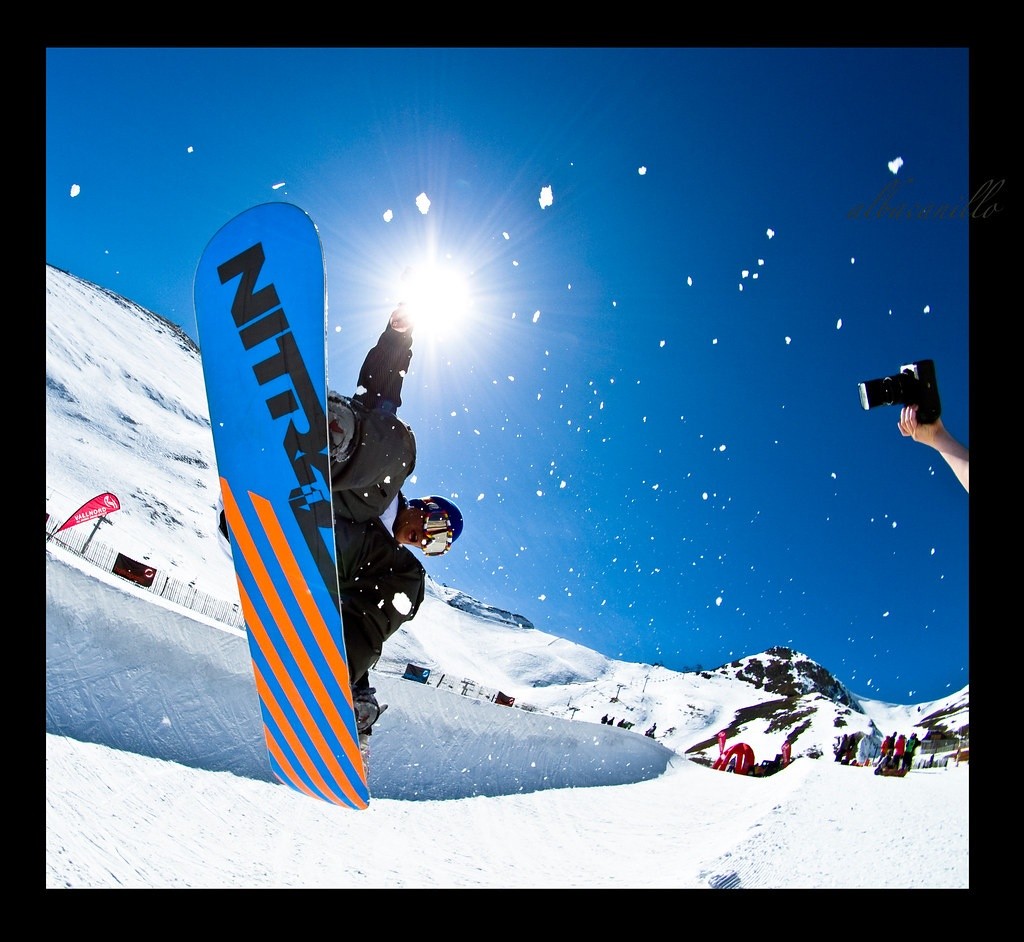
[421,498,452,557]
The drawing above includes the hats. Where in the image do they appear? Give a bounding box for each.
[409,496,463,544]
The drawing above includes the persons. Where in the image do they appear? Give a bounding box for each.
[220,305,463,757]
[601,714,657,737]
[726,756,766,778]
[834,731,923,777]
[896,402,969,496]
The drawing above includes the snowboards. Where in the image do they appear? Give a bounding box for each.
[191,200,371,810]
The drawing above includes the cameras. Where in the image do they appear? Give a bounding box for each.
[858,359,941,426]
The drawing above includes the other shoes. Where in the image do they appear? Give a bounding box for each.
[352,688,380,734]
[327,391,356,458]
